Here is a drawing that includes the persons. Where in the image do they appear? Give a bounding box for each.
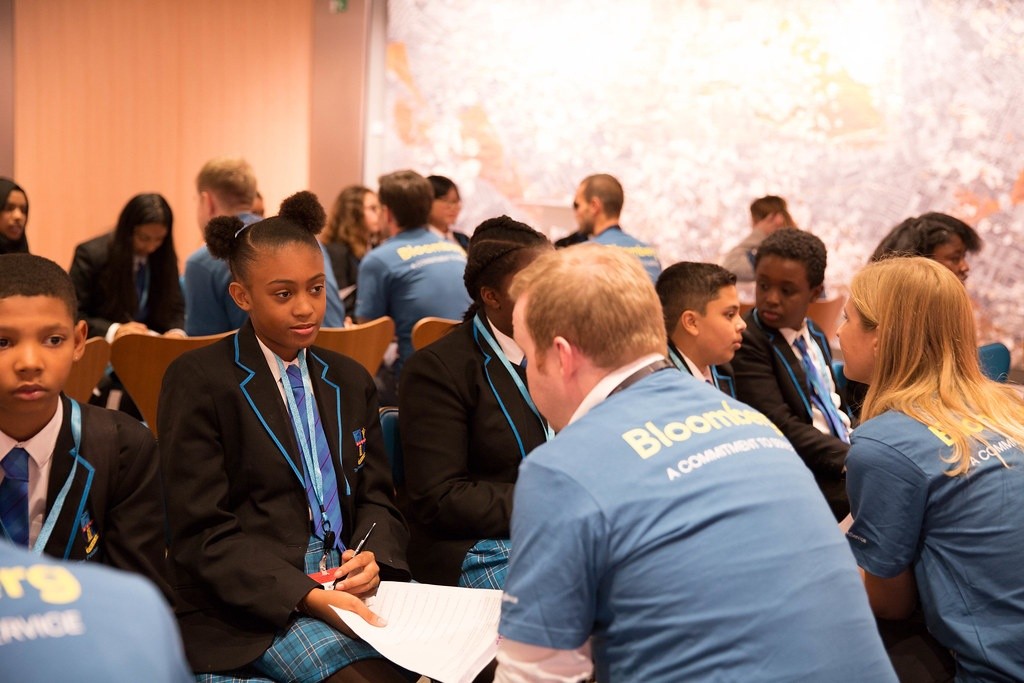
[400,216,565,586]
[0,253,170,601]
[836,256,1024,683]
[0,156,664,424]
[0,539,190,683]
[494,242,900,682]
[654,195,982,554]
[156,192,410,683]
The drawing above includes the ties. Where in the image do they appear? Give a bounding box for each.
[135,262,145,299]
[793,336,848,443]
[0,448,30,549]
[280,364,343,550]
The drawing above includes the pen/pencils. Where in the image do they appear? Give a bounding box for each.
[333,521,376,587]
[126,313,134,321]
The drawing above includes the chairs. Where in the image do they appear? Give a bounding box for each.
[411,316,462,350]
[60,336,110,405]
[108,329,238,442]
[738,294,846,344]
[312,316,396,378]
